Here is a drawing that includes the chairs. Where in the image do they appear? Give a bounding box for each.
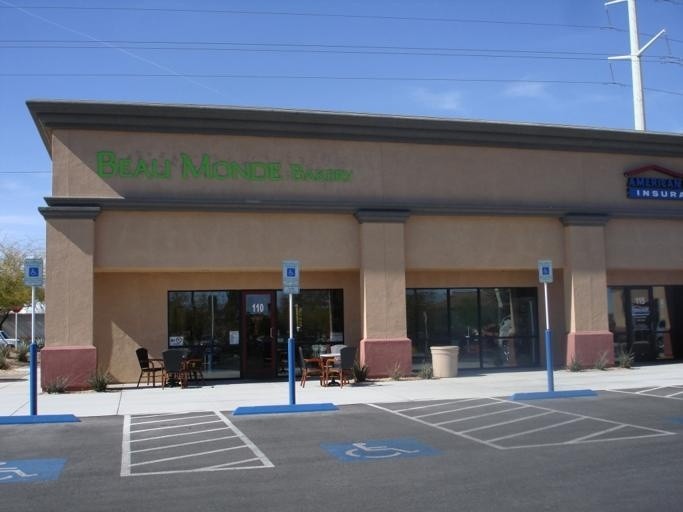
[135,343,207,390]
[298,344,356,388]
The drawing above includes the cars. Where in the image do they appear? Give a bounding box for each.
[173,331,220,354]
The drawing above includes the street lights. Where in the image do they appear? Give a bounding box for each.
[11,301,23,350]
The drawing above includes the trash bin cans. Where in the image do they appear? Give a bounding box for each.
[430,346,460,378]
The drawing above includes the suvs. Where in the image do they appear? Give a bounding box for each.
[0,331,21,351]
